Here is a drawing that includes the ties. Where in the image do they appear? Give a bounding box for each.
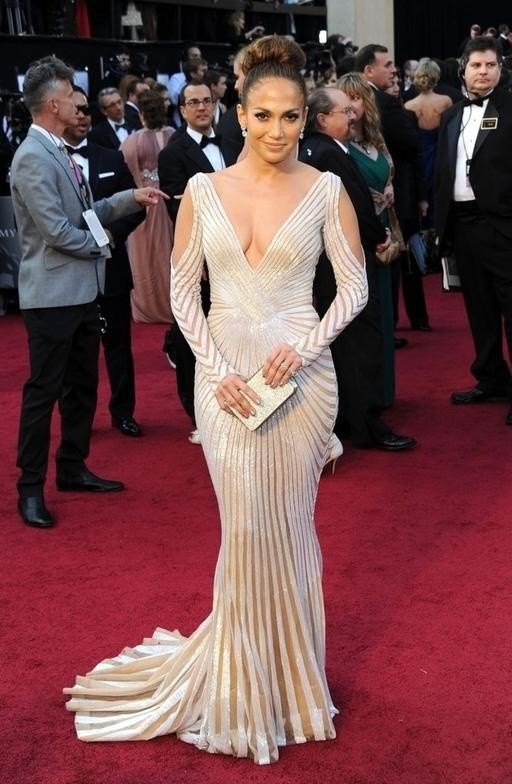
[60,146,88,204]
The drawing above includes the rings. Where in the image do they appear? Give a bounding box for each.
[289,368,298,378]
[281,363,288,370]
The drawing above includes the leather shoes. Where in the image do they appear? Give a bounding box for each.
[355,433,416,451]
[55,471,125,493]
[112,416,141,438]
[451,387,502,404]
[17,495,53,529]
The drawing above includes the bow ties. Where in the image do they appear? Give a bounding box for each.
[116,121,128,132]
[459,97,484,107]
[200,133,223,148]
[65,144,89,158]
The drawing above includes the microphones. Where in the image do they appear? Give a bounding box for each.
[457,66,480,96]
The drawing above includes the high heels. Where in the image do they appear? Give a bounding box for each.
[325,433,343,475]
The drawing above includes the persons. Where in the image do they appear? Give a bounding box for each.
[62,81,150,437]
[94,12,511,453]
[62,29,369,773]
[8,55,174,529]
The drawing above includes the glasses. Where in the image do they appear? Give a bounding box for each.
[75,104,91,116]
[185,99,213,107]
[329,105,353,116]
